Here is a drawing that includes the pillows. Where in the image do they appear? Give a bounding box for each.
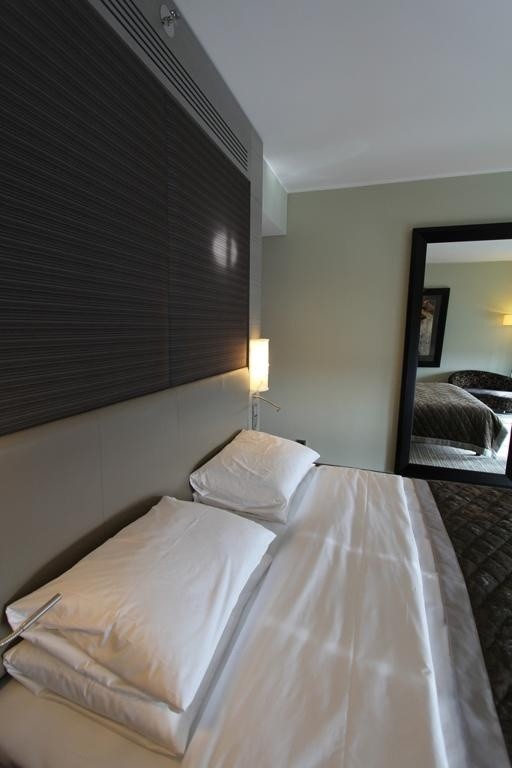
[187,425,321,525]
[3,492,279,761]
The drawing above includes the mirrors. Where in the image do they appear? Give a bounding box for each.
[393,221,512,491]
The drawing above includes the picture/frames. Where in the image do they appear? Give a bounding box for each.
[418,285,450,368]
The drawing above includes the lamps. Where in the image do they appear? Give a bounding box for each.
[244,336,281,432]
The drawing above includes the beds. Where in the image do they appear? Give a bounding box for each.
[0,427,510,768]
[411,382,495,458]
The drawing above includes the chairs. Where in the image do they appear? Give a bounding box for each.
[448,370,512,417]
